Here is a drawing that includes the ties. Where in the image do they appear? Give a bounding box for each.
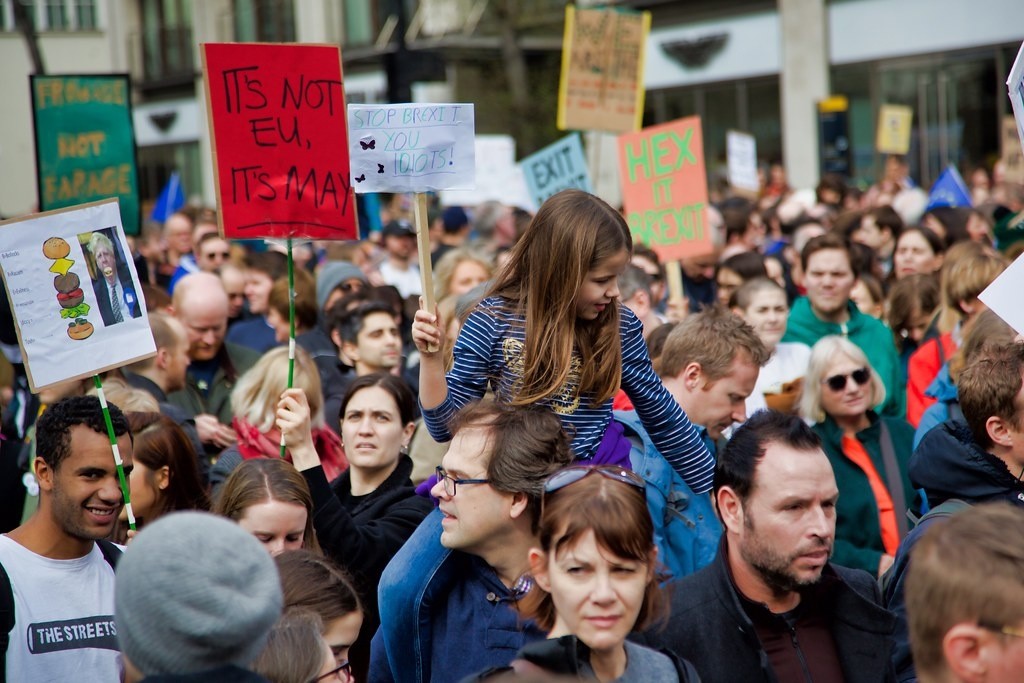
[110,285,124,324]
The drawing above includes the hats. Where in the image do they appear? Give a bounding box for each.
[387,219,416,237]
[314,260,371,317]
[114,510,283,679]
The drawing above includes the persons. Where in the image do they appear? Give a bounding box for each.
[0,151,1023,682]
[112,511,283,683]
[276,549,363,672]
[367,401,570,683]
[511,465,700,683]
[251,614,355,683]
[906,505,1024,683]
[649,409,889,683]
[87,232,142,327]
[0,395,139,683]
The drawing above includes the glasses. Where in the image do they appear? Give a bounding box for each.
[310,659,351,683]
[435,466,492,497]
[821,369,872,392]
[542,465,647,504]
[335,283,367,294]
[208,252,231,262]
